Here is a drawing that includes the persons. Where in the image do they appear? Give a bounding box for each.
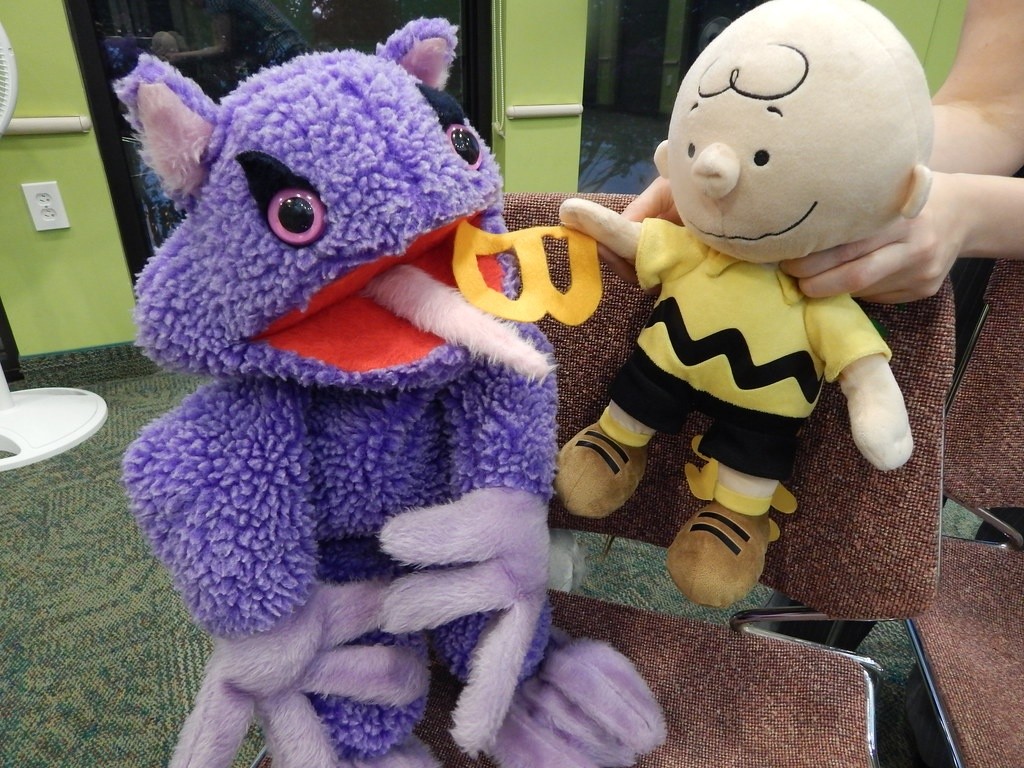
[93,19,193,83]
[598,1,1024,306]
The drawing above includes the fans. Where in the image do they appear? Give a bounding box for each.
[0,25,110,471]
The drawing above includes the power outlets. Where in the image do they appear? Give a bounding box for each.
[20,182,72,232]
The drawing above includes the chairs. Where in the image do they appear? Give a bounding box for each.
[252,191,1024,768]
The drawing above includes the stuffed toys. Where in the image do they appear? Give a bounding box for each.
[554,1,938,610]
[111,12,671,768]
